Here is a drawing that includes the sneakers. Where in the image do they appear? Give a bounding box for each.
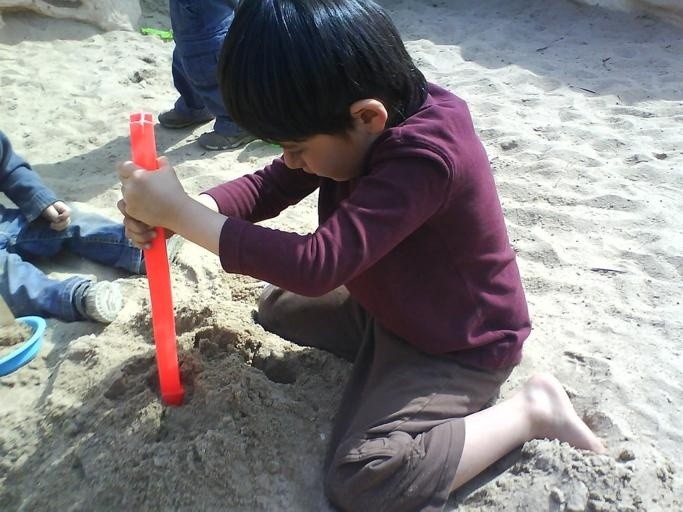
[158,108,213,128]
[140,233,184,274]
[199,132,261,150]
[86,281,122,323]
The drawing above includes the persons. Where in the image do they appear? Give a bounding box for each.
[116,1,605,512]
[157,2,263,149]
[0,132,187,326]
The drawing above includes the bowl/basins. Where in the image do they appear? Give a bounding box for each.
[0,314,46,377]
